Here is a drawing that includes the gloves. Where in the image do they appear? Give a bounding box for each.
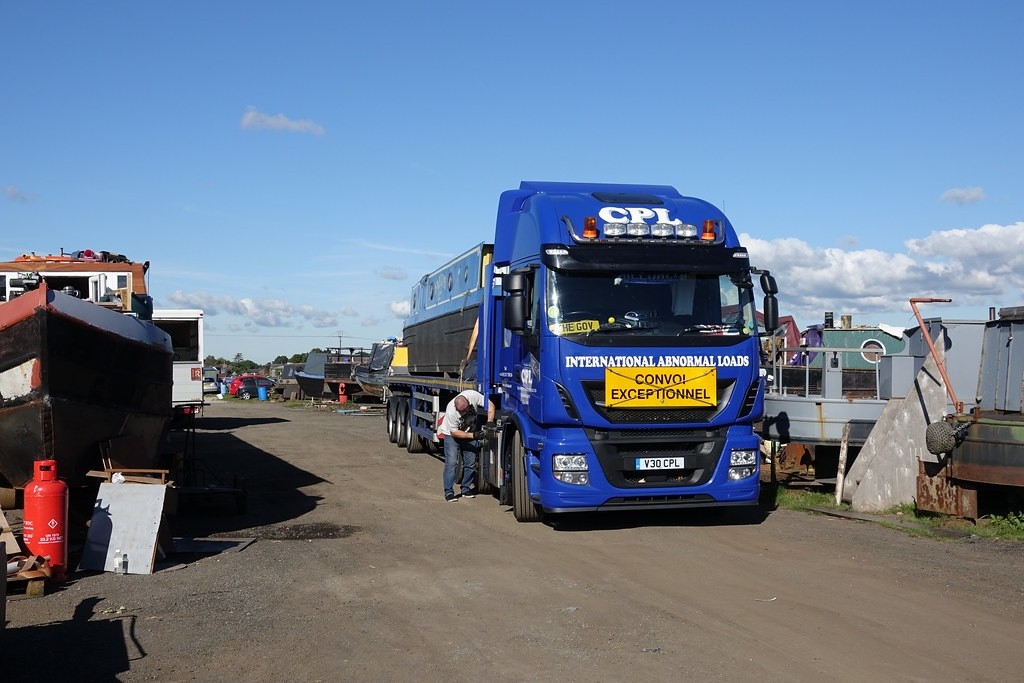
[473,431,484,440]
[486,422,496,428]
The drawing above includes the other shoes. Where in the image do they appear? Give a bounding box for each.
[445,494,458,502]
[461,491,475,498]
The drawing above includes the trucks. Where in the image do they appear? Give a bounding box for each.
[149,309,211,430]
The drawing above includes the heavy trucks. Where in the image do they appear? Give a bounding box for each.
[350,180,780,523]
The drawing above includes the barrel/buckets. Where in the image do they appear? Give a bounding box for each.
[257,386,267,400]
[841,316,851,328]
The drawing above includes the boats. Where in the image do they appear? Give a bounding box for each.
[293,330,411,405]
[0,248,181,517]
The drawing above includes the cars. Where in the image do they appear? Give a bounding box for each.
[203,365,277,401]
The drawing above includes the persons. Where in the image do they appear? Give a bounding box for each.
[441,390,496,502]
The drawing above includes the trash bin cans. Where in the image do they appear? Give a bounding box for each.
[258,385,268,401]
